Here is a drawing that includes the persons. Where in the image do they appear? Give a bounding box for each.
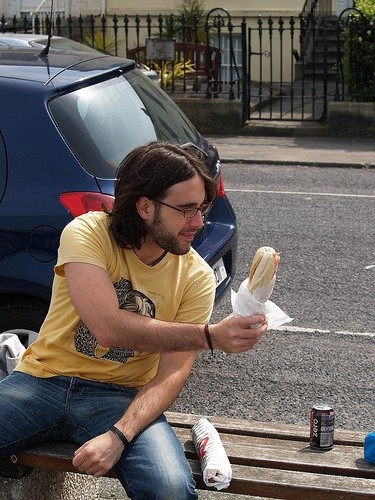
[0,141,269,500]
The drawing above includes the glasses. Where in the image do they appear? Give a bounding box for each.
[150,200,213,219]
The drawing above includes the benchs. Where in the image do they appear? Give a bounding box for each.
[0,410,375,500]
[126,43,220,99]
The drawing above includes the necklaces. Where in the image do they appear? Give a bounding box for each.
[149,251,167,266]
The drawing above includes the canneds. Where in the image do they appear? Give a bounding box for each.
[310,404,336,451]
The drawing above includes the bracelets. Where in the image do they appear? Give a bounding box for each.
[205,324,213,351]
[109,425,129,447]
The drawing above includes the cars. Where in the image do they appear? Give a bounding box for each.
[0,33,239,353]
